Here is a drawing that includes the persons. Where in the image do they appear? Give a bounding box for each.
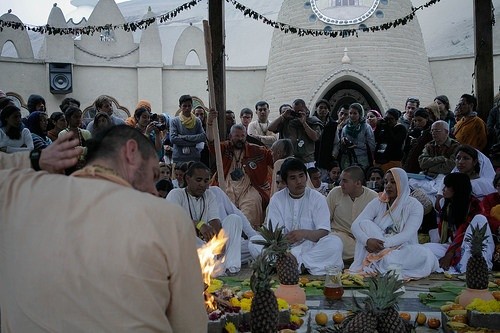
[249,159,344,275]
[0,124,209,333]
[0,92,500,243]
[423,172,495,274]
[325,166,379,260]
[164,161,242,274]
[349,167,440,280]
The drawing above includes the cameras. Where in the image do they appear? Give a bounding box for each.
[155,123,166,131]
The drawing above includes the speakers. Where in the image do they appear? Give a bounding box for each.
[48,63,73,94]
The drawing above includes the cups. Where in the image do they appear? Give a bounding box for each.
[324,265,344,302]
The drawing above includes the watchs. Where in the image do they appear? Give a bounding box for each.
[29,149,41,171]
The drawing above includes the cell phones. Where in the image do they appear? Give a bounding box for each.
[290,111,302,118]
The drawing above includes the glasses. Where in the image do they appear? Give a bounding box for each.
[40,118,49,125]
[382,116,395,122]
[429,128,446,134]
[241,115,252,121]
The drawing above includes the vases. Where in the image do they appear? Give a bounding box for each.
[274,284,306,306]
[459,287,496,308]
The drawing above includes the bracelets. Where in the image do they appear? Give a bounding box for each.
[196,221,204,229]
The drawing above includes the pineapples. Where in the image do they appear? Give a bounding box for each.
[340,271,412,333]
[465,223,489,289]
[256,218,300,285]
[250,257,280,333]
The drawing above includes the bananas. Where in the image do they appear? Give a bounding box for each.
[441,305,469,329]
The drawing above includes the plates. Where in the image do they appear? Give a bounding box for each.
[342,285,371,289]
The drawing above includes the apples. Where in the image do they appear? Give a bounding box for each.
[452,315,465,324]
[492,291,500,301]
[451,304,464,311]
[494,279,500,285]
[299,277,309,285]
[400,312,440,328]
[315,311,355,325]
[244,290,254,298]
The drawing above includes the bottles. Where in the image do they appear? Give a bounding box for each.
[459,287,495,307]
[273,282,306,307]
